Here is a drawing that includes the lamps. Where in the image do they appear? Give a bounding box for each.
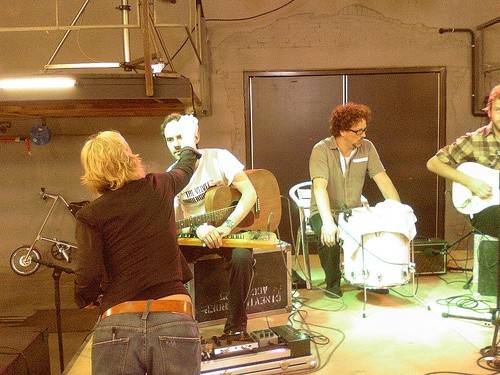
[0,75,77,89]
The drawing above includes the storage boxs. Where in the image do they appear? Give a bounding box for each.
[474,232,500,302]
[184,238,291,326]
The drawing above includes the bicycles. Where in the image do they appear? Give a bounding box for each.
[9,188,90,277]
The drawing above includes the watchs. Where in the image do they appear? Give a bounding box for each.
[225,219,235,230]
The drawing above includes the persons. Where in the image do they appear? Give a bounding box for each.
[308,101,401,299]
[426,85,500,325]
[74,132,201,375]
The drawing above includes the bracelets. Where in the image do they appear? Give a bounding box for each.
[189,224,203,238]
[160,113,258,340]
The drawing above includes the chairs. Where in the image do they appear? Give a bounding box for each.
[289,180,370,288]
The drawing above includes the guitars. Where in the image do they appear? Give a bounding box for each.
[175,169,281,234]
[452,162,500,215]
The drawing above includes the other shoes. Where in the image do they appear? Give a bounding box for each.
[226,327,246,335]
[358,287,389,294]
[325,283,342,298]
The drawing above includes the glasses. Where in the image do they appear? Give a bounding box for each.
[348,127,368,136]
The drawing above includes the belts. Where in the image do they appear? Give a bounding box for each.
[99,301,193,321]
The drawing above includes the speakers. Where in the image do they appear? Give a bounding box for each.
[409,239,447,275]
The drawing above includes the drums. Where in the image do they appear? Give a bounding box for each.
[340,206,411,289]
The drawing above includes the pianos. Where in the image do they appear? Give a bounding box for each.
[177,230,277,251]
